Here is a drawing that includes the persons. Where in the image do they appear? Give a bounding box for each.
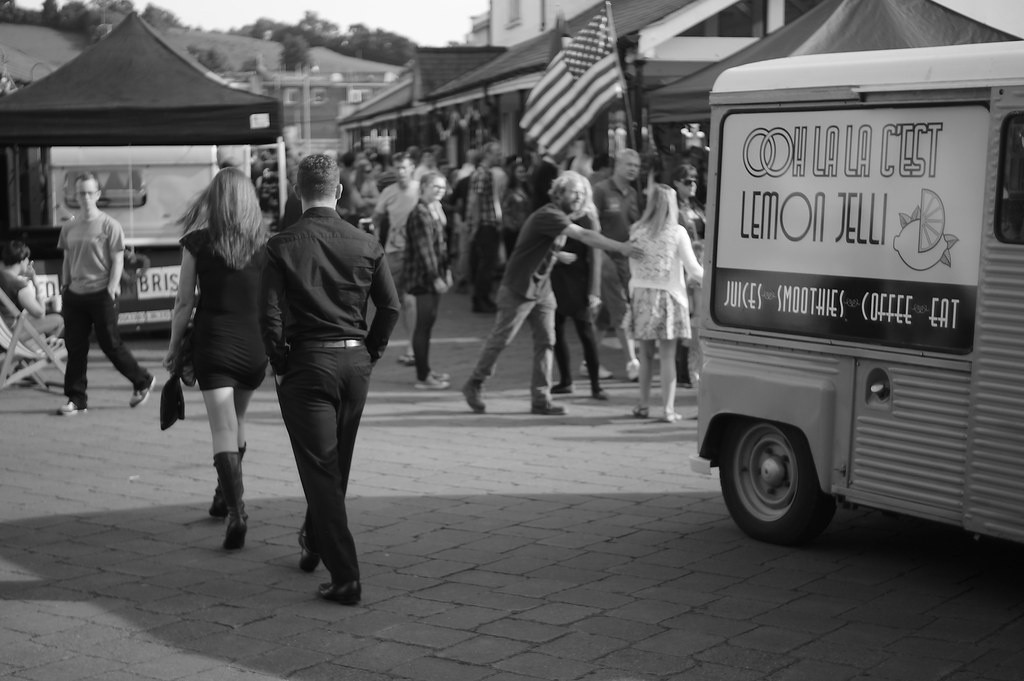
[163,167,274,550]
[257,154,400,606]
[58,174,155,416]
[0,240,65,385]
[287,139,723,425]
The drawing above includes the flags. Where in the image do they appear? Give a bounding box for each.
[519,5,627,163]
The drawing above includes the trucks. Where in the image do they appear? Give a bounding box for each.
[697,41,1024,547]
[49,146,251,331]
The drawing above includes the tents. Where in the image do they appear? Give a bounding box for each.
[0,12,289,237]
[644,0,1024,128]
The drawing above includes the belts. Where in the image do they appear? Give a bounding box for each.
[289,339,367,349]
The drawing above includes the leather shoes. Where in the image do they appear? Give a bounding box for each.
[319,574,362,603]
[300,546,320,572]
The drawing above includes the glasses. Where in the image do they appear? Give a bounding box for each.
[678,179,698,186]
[74,190,101,197]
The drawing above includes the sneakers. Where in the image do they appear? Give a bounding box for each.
[57,402,87,416]
[579,360,614,379]
[626,359,642,381]
[129,374,155,407]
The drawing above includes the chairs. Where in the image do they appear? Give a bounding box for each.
[0,286,65,390]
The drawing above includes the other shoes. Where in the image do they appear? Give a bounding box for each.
[398,350,416,365]
[415,370,451,389]
[471,300,498,313]
[532,401,564,415]
[592,389,608,399]
[550,383,573,394]
[463,382,486,412]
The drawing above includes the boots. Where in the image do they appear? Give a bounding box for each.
[213,451,248,550]
[208,442,247,521]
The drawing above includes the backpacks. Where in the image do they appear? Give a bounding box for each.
[469,225,506,281]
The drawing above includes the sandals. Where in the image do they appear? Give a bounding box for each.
[662,410,682,423]
[632,405,649,419]
